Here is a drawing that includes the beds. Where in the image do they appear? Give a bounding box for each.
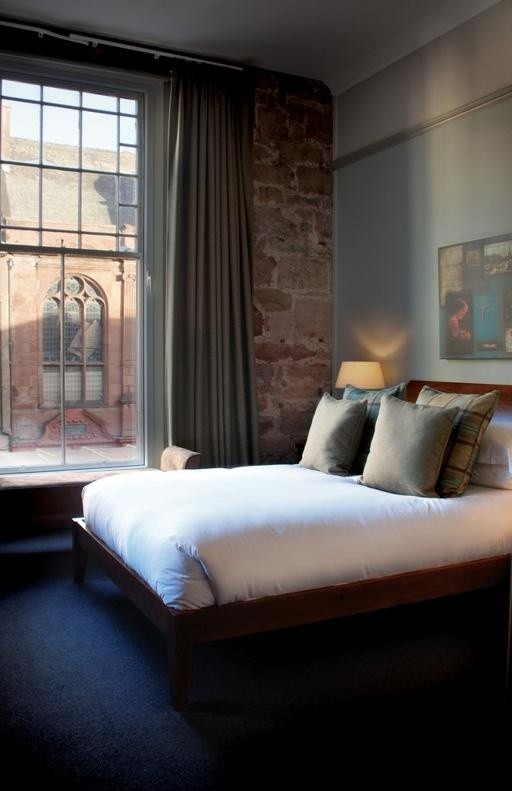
[71,378,512,711]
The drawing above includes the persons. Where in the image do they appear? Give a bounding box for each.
[447,297,470,351]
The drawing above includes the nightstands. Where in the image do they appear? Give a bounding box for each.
[290,431,308,463]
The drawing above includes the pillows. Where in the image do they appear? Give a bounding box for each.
[355,393,460,497]
[416,385,502,498]
[342,380,409,475]
[298,392,369,477]
[469,419,512,490]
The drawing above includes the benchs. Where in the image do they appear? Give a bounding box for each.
[0,444,202,544]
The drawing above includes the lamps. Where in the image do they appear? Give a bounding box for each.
[336,360,386,390]
